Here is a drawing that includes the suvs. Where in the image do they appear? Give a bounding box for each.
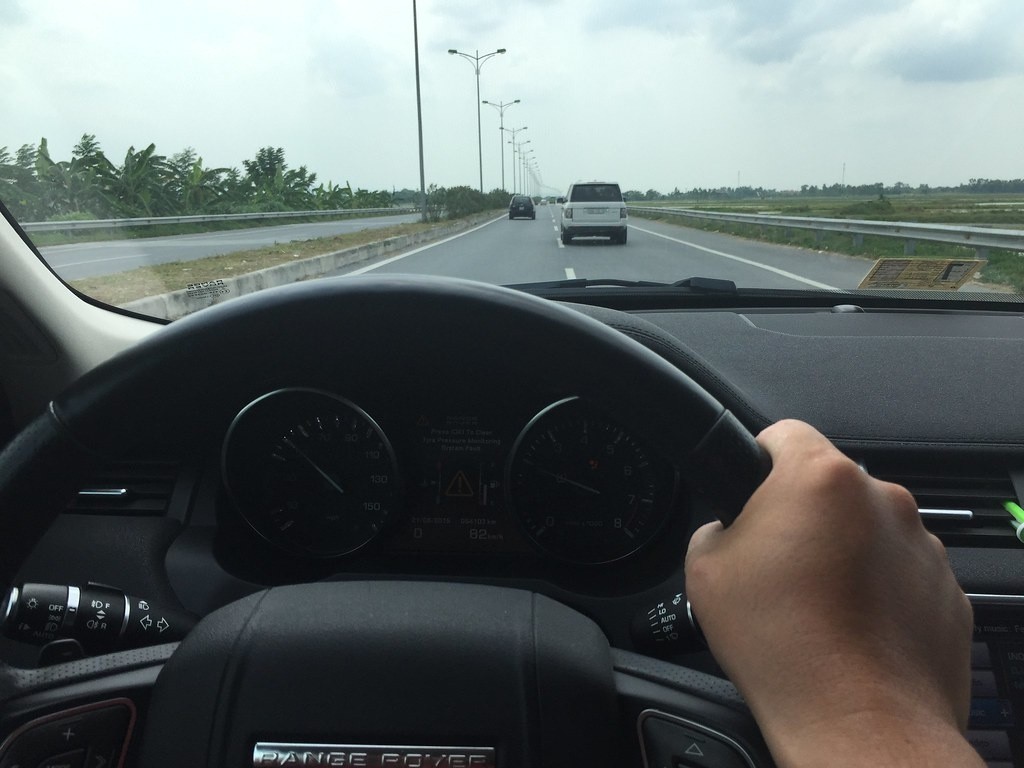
[508,195,536,221]
[557,180,628,245]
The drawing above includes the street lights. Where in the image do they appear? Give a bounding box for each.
[482,99,520,191]
[448,48,506,192]
[499,126,543,197]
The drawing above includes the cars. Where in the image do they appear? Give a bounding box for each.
[535,196,563,206]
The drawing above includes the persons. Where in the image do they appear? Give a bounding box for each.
[685,417,987,768]
